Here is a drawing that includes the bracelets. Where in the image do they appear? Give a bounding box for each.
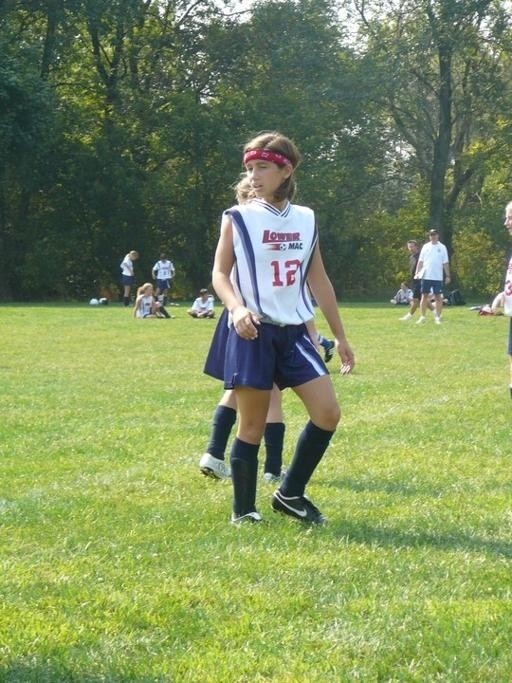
[445,275,450,278]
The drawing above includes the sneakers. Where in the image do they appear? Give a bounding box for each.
[262,470,286,487]
[270,488,325,523]
[231,511,263,529]
[324,340,335,363]
[435,315,441,324]
[399,312,413,321]
[415,316,426,324]
[199,452,232,480]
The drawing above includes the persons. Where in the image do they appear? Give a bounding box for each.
[414,228,452,324]
[398,238,443,321]
[151,252,176,305]
[479,200,512,397]
[200,175,287,481]
[390,281,415,305]
[316,332,335,362]
[186,288,216,319]
[212,133,355,526]
[132,282,176,320]
[120,249,139,306]
